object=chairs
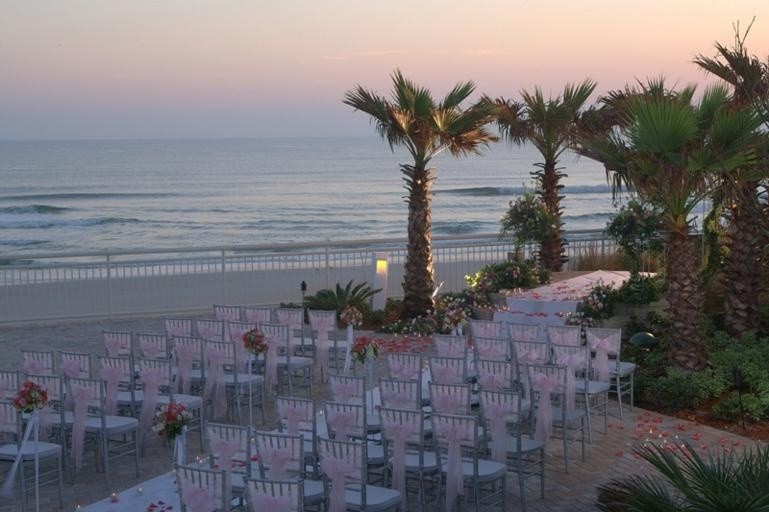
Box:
[355,318,643,512]
[0,299,354,511]
